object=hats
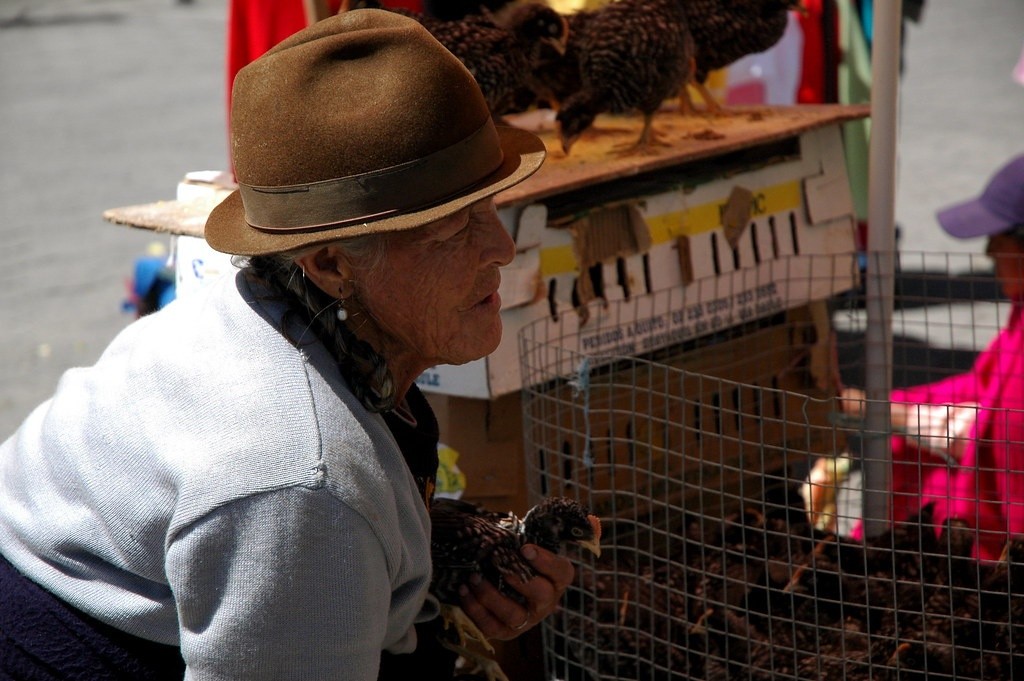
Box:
[937,155,1024,239]
[203,8,547,257]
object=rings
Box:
[509,612,529,630]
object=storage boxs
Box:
[425,300,854,527]
[171,123,866,399]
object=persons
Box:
[0,8,573,681]
[843,153,1024,567]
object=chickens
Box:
[339,1,1024,681]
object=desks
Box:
[96,99,870,243]
